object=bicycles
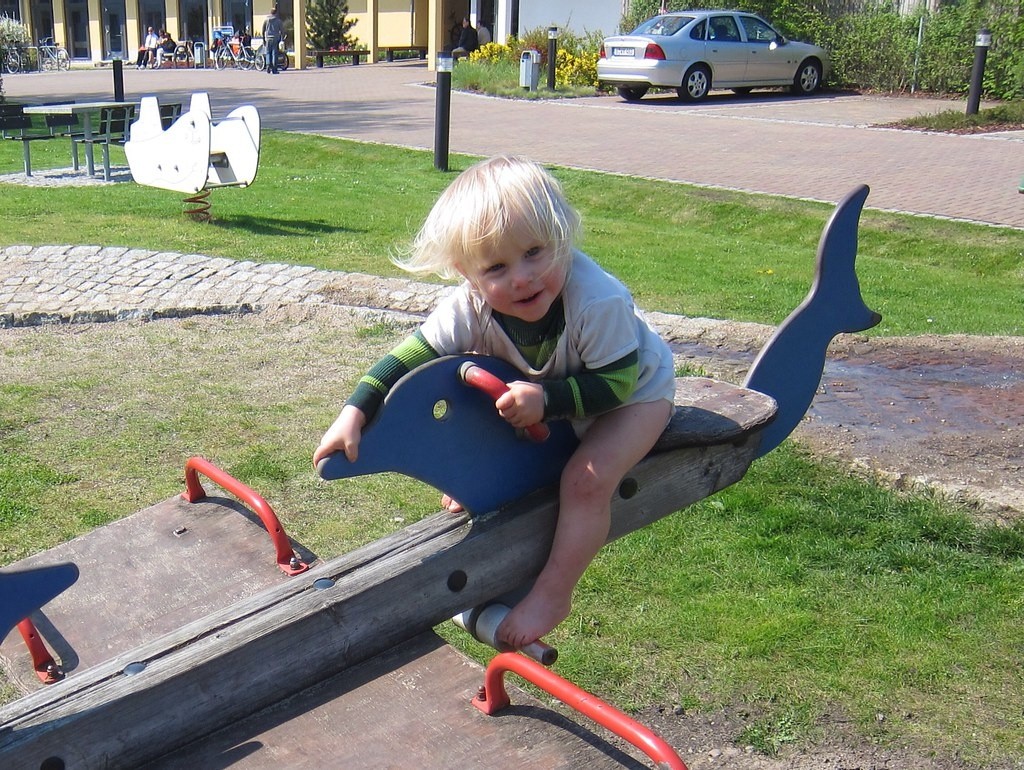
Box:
[0,32,32,75]
[38,35,72,72]
[214,32,256,71]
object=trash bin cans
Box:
[518,50,542,93]
[193,41,207,69]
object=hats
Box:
[162,33,170,38]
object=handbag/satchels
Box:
[279,41,285,53]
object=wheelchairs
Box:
[254,32,289,71]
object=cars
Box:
[596,10,830,105]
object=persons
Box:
[261,8,286,74]
[451,17,478,59]
[476,20,491,52]
[135,27,171,70]
[314,157,677,646]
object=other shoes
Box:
[267,66,270,73]
[272,70,279,74]
[140,65,145,70]
[135,65,139,69]
